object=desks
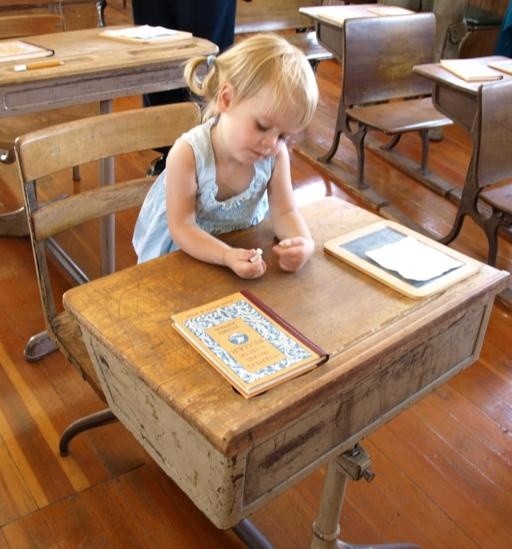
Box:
[298,4,415,61]
[375,55,510,244]
[0,24,219,284]
[62,198,510,548]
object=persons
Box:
[130,29,324,281]
[129,1,253,177]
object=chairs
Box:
[458,15,511,58]
[440,83,511,267]
[234,0,338,62]
[0,0,80,238]
[316,15,456,210]
[15,103,202,457]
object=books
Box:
[319,8,378,25]
[170,287,331,401]
[0,39,55,63]
[439,57,504,82]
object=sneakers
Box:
[145,155,165,175]
